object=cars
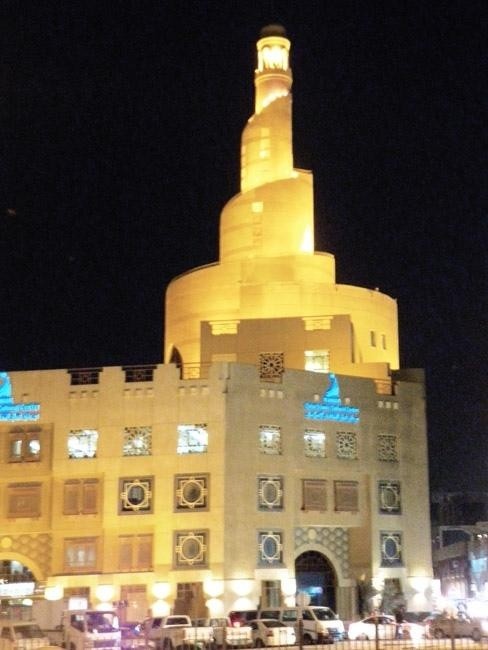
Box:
[243,618,296,648]
[117,625,156,649]
[346,612,487,644]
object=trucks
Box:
[190,615,254,650]
[134,614,216,650]
[29,592,124,649]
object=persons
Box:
[395,607,405,639]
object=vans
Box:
[0,617,49,650]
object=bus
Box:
[227,602,346,646]
